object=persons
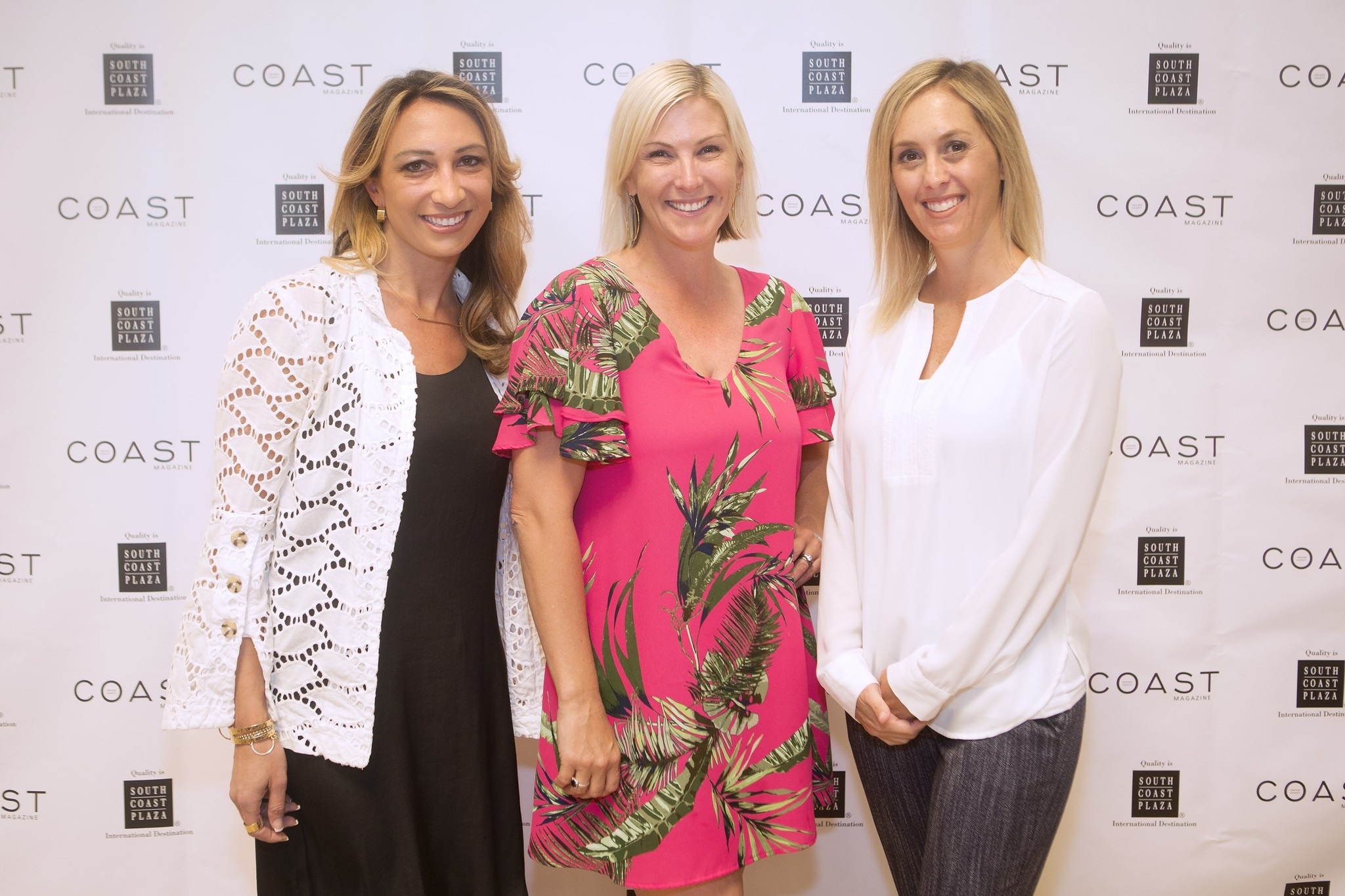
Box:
[814,60,1122,896]
[492,61,835,896]
[161,71,546,896]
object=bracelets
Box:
[219,718,283,755]
[813,532,822,544]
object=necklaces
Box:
[380,274,462,327]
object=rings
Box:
[571,777,590,788]
[244,819,261,833]
[798,554,814,568]
